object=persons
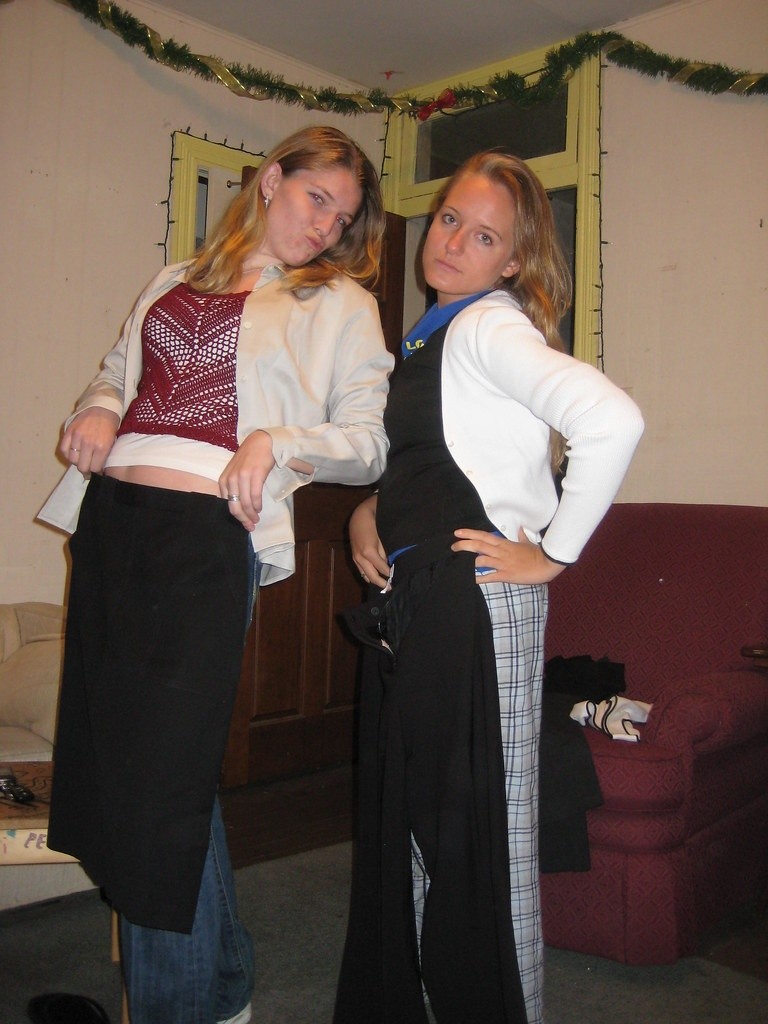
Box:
[36,122,397,1024]
[332,146,644,1024]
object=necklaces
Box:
[242,265,266,274]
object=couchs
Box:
[539,502,768,970]
[0,601,66,761]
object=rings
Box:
[227,494,239,502]
[361,574,366,578]
[70,447,80,451]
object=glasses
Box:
[0,773,37,805]
